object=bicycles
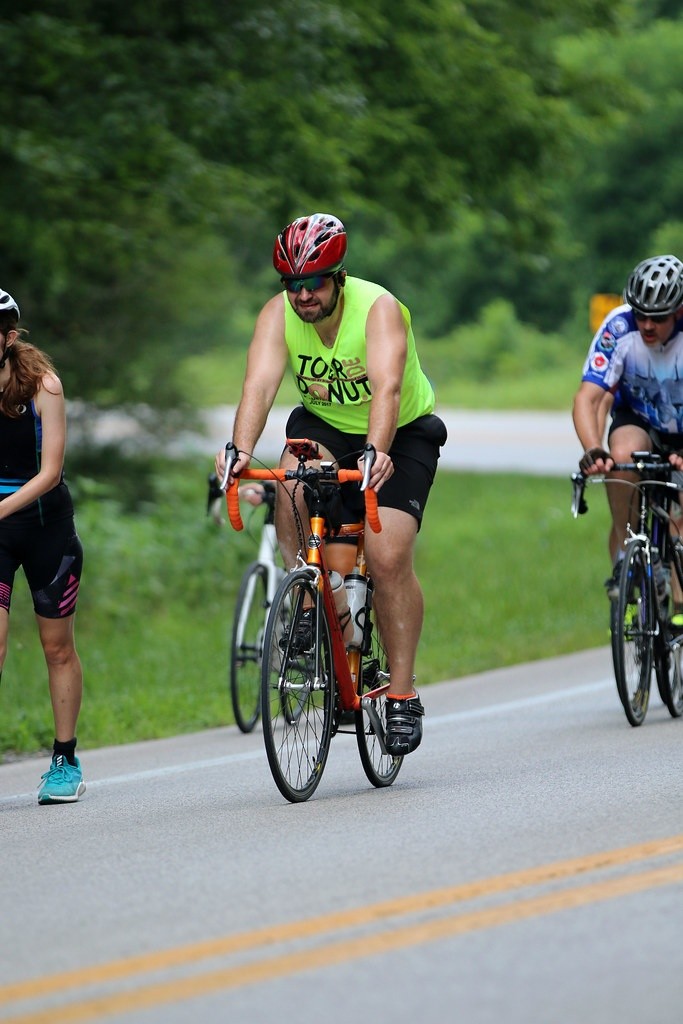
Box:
[203,470,320,737]
[566,450,683,727]
[218,437,406,805]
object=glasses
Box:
[280,273,333,292]
[635,312,673,322]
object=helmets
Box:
[626,255,683,316]
[0,289,20,325]
[274,213,347,278]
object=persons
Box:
[572,255,683,630]
[240,476,325,653]
[0,286,86,805]
[215,213,448,755]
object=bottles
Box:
[651,552,666,596]
[324,572,355,642]
[341,573,368,646]
[661,562,671,594]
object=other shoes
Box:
[606,561,633,598]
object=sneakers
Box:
[385,689,425,755]
[37,756,87,802]
[279,610,316,650]
